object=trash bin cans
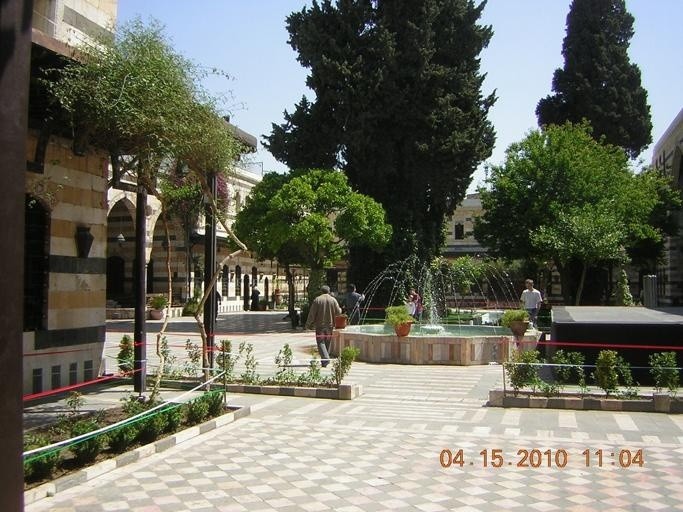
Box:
[268,302,275,310]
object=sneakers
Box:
[321,361,330,367]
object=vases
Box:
[336,313,348,329]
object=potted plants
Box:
[257,299,266,310]
[499,309,531,336]
[385,305,415,337]
[150,296,167,319]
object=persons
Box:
[520,279,543,327]
[347,283,365,325]
[306,285,342,367]
[402,288,424,321]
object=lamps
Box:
[75,225,94,259]
